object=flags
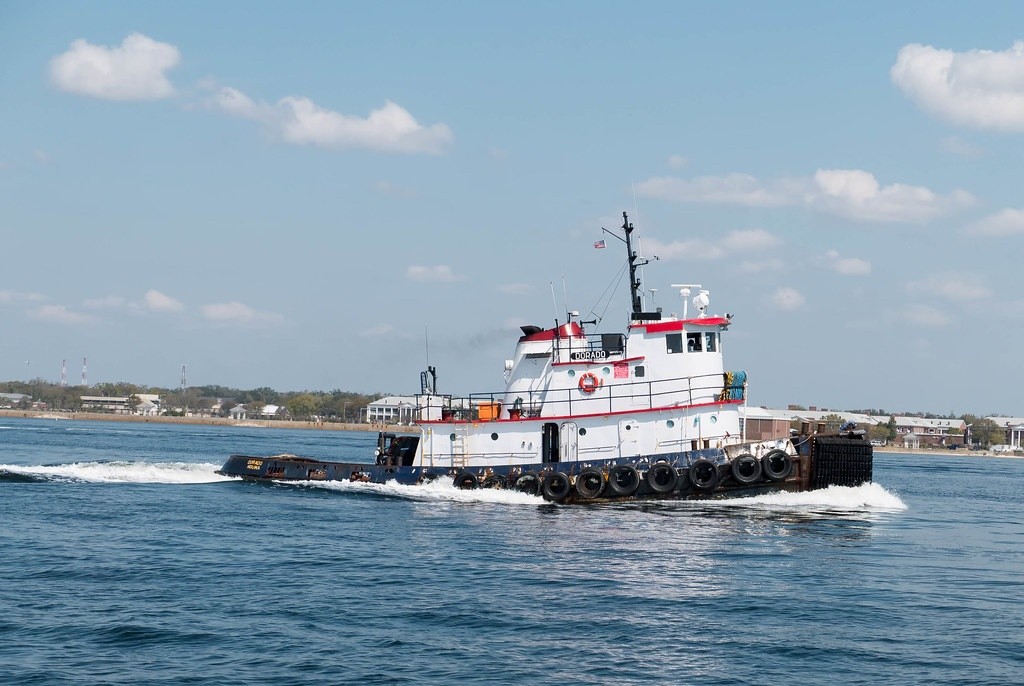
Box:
[592,240,606,249]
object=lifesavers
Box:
[578,372,599,393]
[451,471,571,501]
[688,458,720,491]
[575,464,640,500]
[646,462,679,495]
[730,448,793,485]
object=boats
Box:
[212,210,874,510]
